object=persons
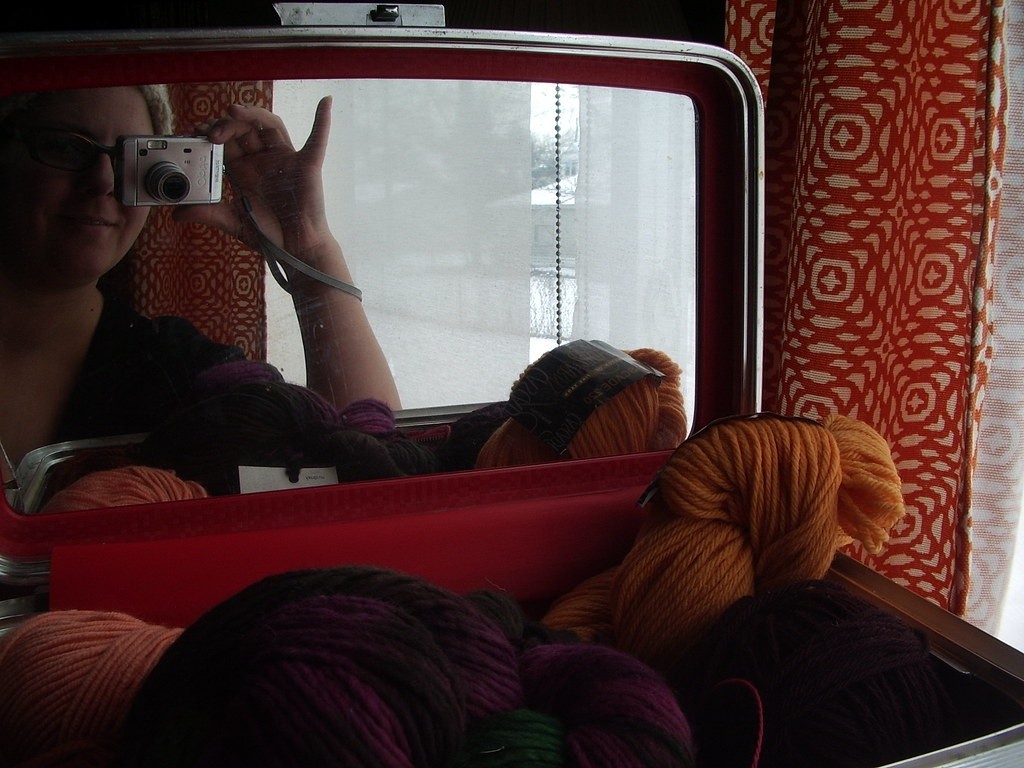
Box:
[1,83,401,465]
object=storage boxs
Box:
[0,2,1024,768]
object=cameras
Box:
[116,134,225,206]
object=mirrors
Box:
[2,78,696,515]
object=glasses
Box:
[9,123,117,173]
